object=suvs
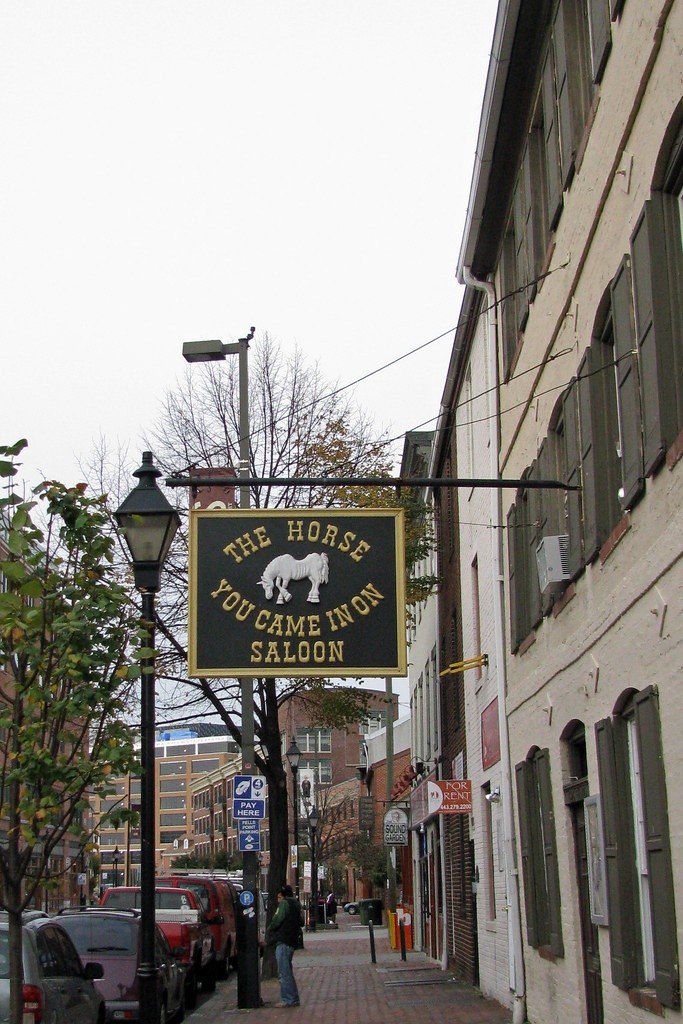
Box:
[51,906,186,1024]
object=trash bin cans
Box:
[387,910,413,949]
[357,899,383,925]
[308,898,327,924]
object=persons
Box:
[266,885,304,1007]
[324,890,338,924]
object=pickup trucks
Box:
[87,887,225,1011]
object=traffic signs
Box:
[238,819,261,852]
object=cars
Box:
[0,910,110,1024]
[344,901,360,915]
[211,878,241,973]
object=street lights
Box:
[183,326,258,889]
[112,846,121,886]
[284,734,304,903]
[109,450,182,1024]
[308,805,320,933]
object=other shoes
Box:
[274,1000,300,1008]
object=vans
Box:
[154,876,244,981]
